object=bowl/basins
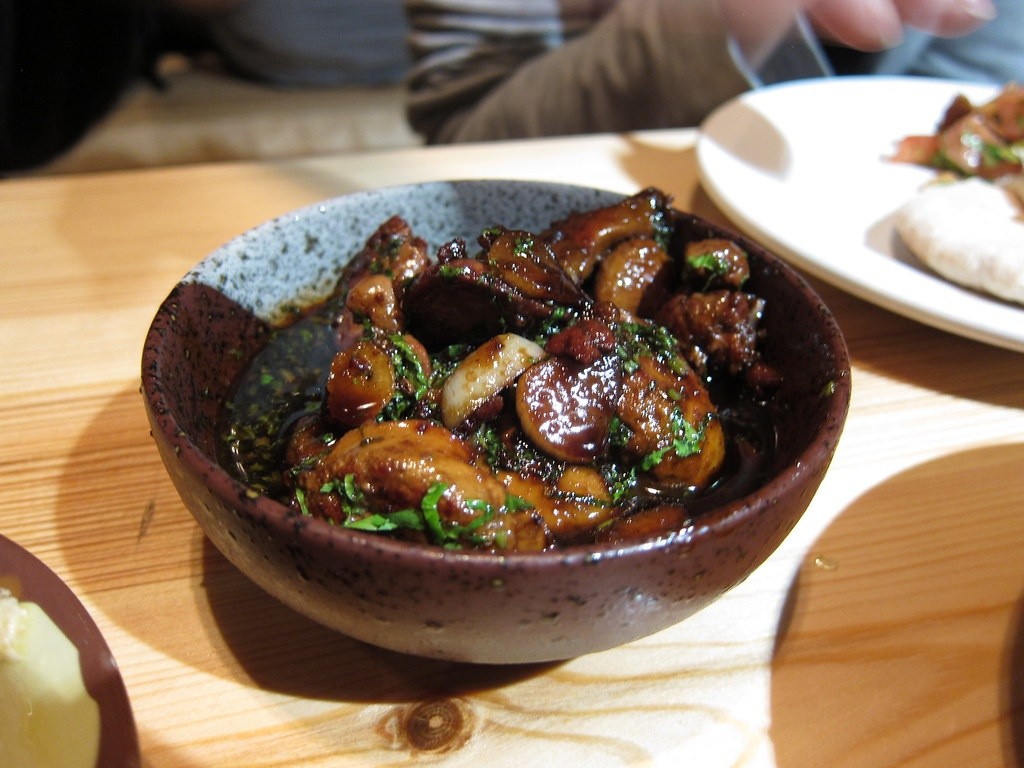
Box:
[140,180,852,666]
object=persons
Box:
[403,0,1024,147]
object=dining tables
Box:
[0,123,1024,768]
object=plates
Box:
[0,534,140,768]
[700,73,1024,354]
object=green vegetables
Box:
[299,318,710,552]
